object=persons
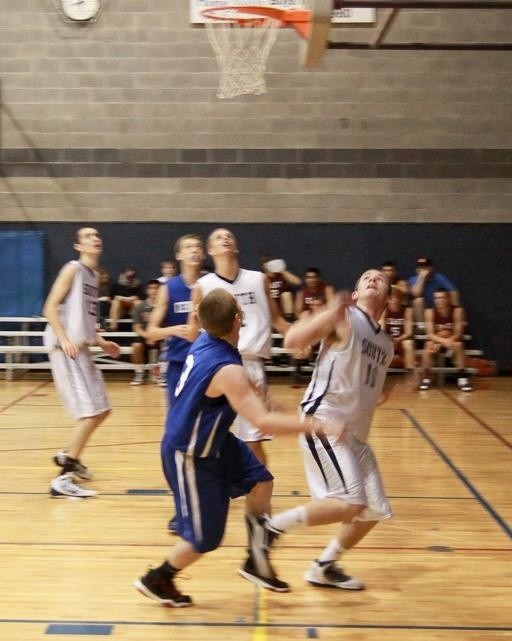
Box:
[131,280,162,385]
[263,255,304,324]
[290,297,326,388]
[106,267,138,332]
[418,288,472,391]
[246,270,392,589]
[156,260,177,386]
[291,269,337,319]
[146,234,211,531]
[94,267,112,333]
[135,290,353,606]
[185,228,290,468]
[42,226,122,500]
[378,288,418,387]
[382,262,410,305]
[407,258,461,335]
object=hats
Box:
[410,256,430,267]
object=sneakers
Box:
[167,516,181,534]
[53,452,92,480]
[237,555,290,592]
[157,374,169,386]
[304,559,364,590]
[130,374,145,386]
[134,569,192,607]
[247,514,275,556]
[458,378,473,391]
[419,376,433,390]
[50,477,98,500]
